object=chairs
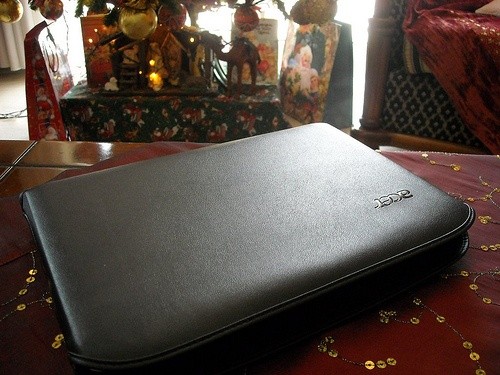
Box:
[347,0,499,155]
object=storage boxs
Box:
[79,13,121,87]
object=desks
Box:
[60,80,283,149]
[0,137,500,375]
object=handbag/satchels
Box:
[278,17,353,129]
[22,20,72,141]
[19,121,474,375]
[229,16,279,85]
